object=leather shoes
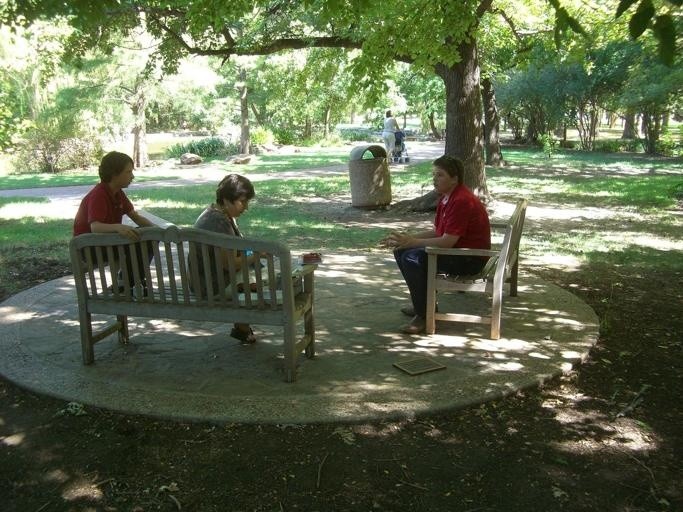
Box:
[399,301,439,333]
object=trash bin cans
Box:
[350,145,392,208]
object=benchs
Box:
[63,226,318,385]
[424,199,528,344]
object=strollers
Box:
[390,131,410,163]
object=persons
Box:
[74,152,160,296]
[382,111,400,163]
[187,174,266,344]
[380,155,491,334]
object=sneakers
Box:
[230,328,256,343]
